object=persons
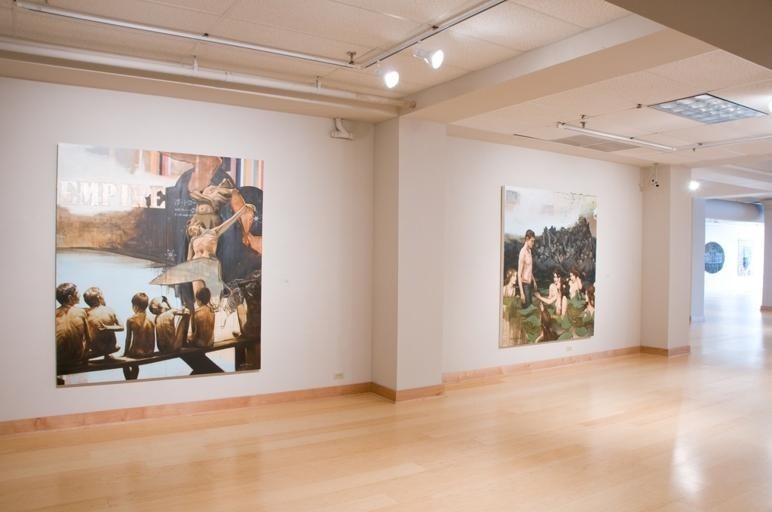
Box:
[81,287,125,363]
[121,292,157,380]
[149,294,191,354]
[503,267,519,347]
[186,203,257,310]
[170,156,255,310]
[517,228,539,307]
[534,267,594,343]
[187,287,216,348]
[56,282,92,385]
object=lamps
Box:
[376,60,399,88]
[413,40,444,69]
